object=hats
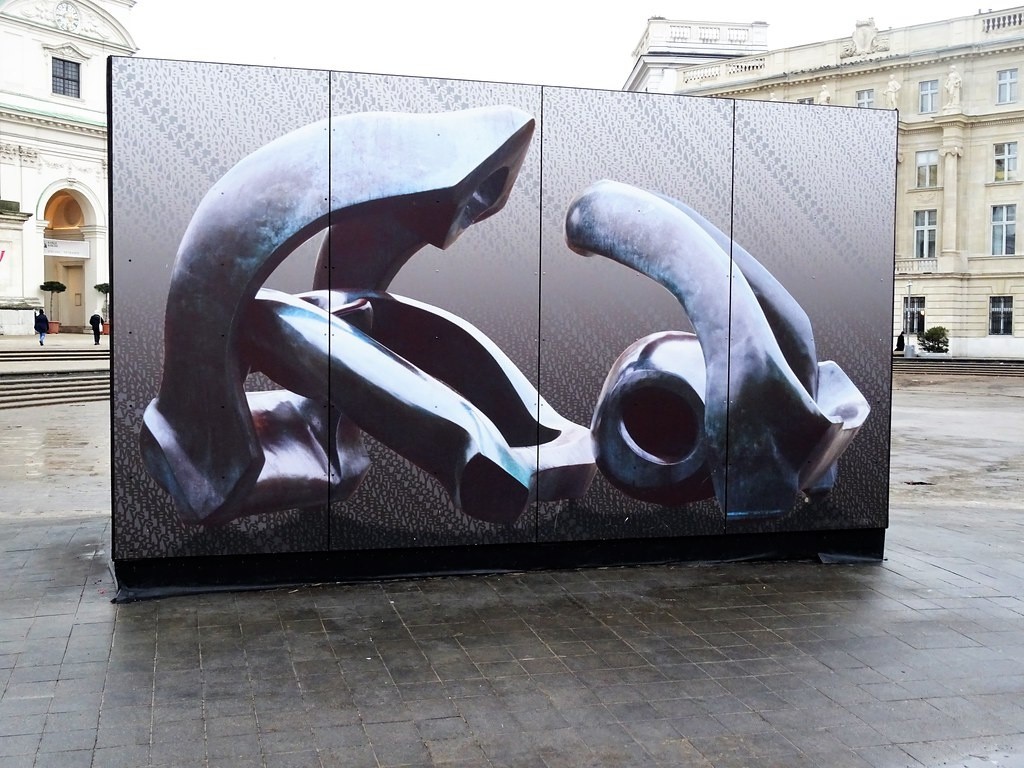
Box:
[39,309,44,313]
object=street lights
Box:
[906,280,913,345]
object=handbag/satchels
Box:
[99,317,103,332]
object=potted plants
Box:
[40,281,67,334]
[94,283,109,335]
[916,326,952,358]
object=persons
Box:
[944,65,962,109]
[90,311,104,345]
[818,85,830,104]
[897,331,904,357]
[769,93,777,101]
[34,309,49,345]
[883,74,901,109]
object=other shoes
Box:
[40,341,43,346]
[95,342,99,345]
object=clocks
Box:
[54,0,80,32]
